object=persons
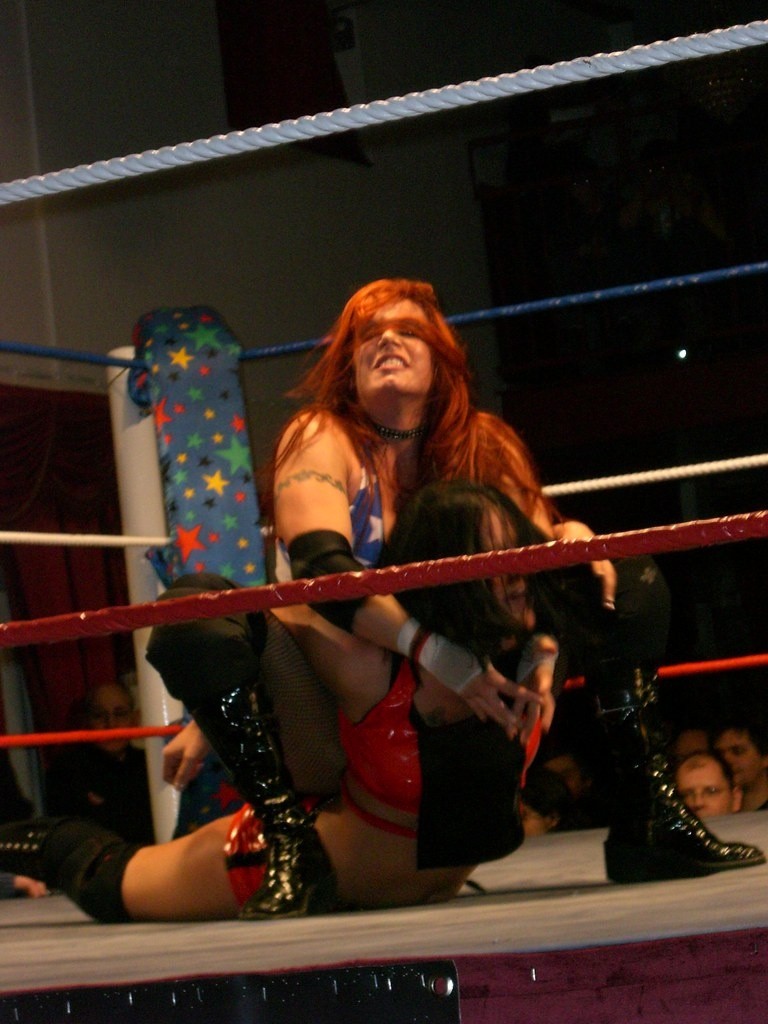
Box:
[0,479,768,923]
[143,276,768,916]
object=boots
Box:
[173,677,338,923]
[577,658,765,886]
[0,814,74,881]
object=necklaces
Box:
[364,419,429,443]
